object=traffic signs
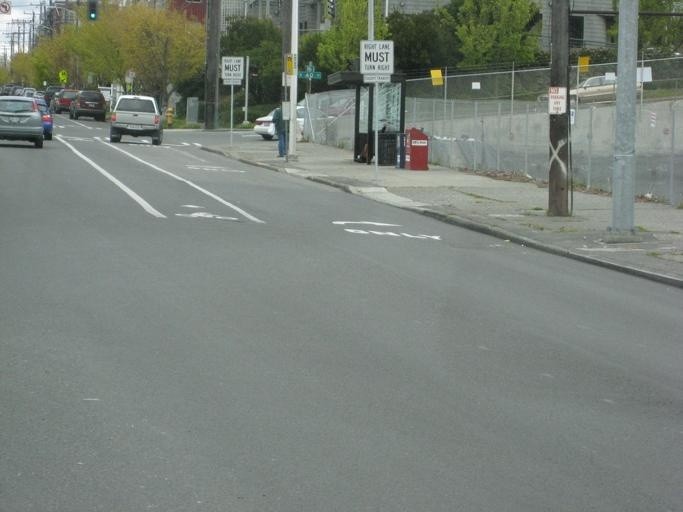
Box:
[298,61,321,80]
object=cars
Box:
[254,105,338,140]
[536,75,643,105]
[109,94,163,145]
[0,83,106,148]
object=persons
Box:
[272,100,290,158]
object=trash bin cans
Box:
[377,130,397,167]
[357,133,375,162]
[403,128,428,170]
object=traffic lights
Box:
[88,2,97,19]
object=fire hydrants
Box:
[165,106,174,129]
